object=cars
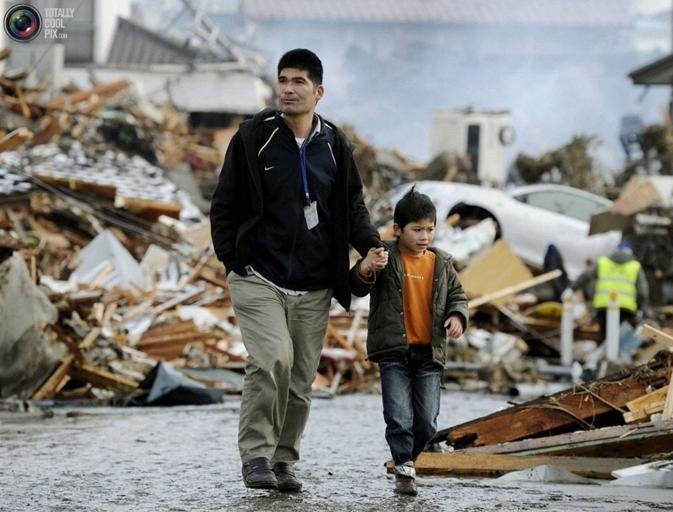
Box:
[375,174,668,304]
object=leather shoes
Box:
[242,457,279,489]
[274,461,302,492]
[392,461,417,495]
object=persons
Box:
[349,183,469,497]
[591,240,651,342]
[651,264,672,329]
[209,45,391,496]
[569,255,598,316]
